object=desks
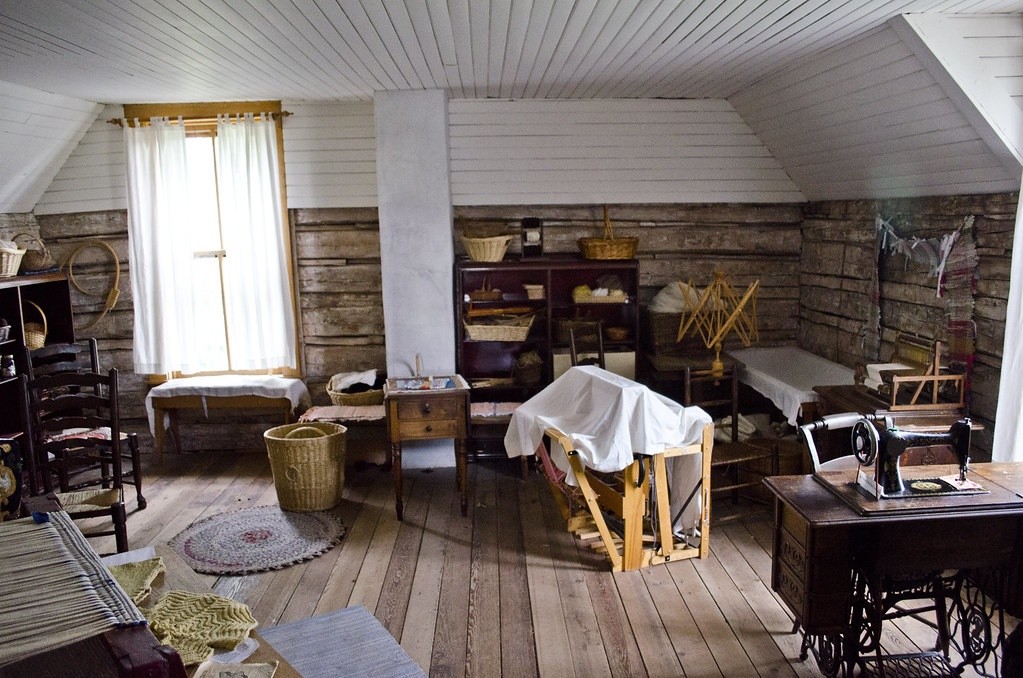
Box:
[384,371,472,523]
[722,344,856,473]
[757,457,1023,678]
[143,373,308,463]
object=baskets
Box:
[553,317,604,343]
[577,203,639,261]
[11,232,50,271]
[458,234,514,263]
[0,243,26,277]
[463,315,535,342]
[21,299,49,351]
[515,360,543,386]
[326,377,385,406]
[571,292,629,303]
[606,326,633,340]
[469,272,502,301]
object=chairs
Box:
[25,335,148,512]
[684,360,780,532]
[14,366,129,554]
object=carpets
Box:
[165,503,348,578]
[256,603,429,678]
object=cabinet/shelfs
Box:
[0,271,81,478]
[454,252,640,468]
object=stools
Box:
[796,407,956,664]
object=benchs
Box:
[100,541,303,678]
[297,401,531,478]
